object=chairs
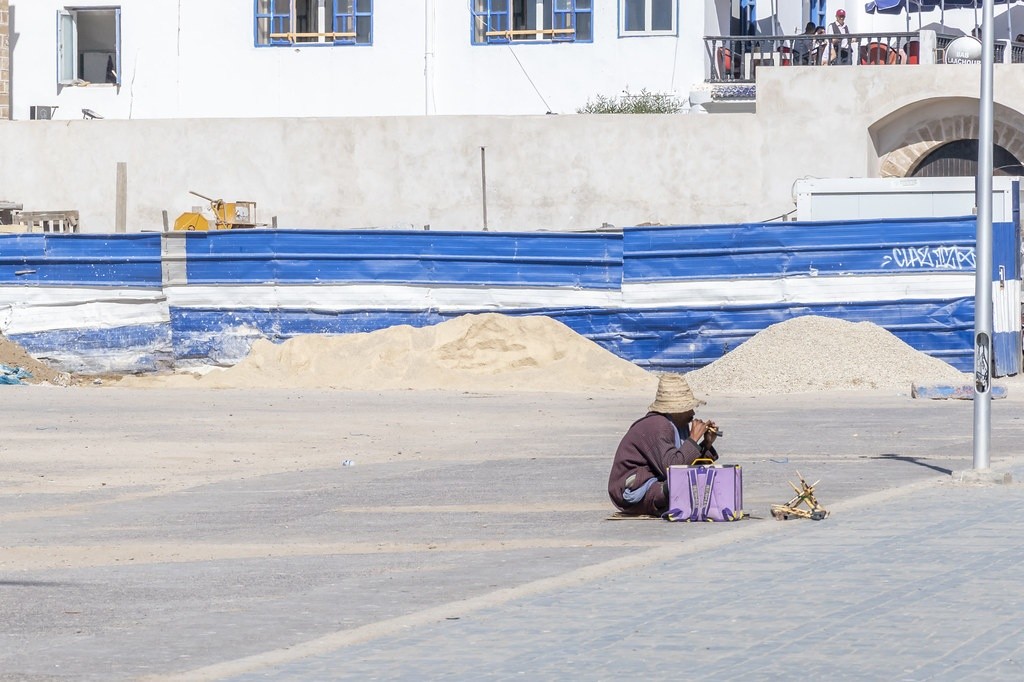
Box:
[860,41,919,64]
[777,46,800,65]
[717,47,742,78]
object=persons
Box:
[792,22,816,66]
[827,9,857,65]
[1016,34,1024,43]
[971,28,982,40]
[608,373,719,517]
[809,26,837,65]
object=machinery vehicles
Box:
[190,190,257,230]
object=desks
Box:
[744,52,794,80]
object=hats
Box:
[650,373,706,413]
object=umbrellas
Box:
[865,0,1024,42]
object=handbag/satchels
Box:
[662,458,744,521]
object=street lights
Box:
[937,34,994,472]
